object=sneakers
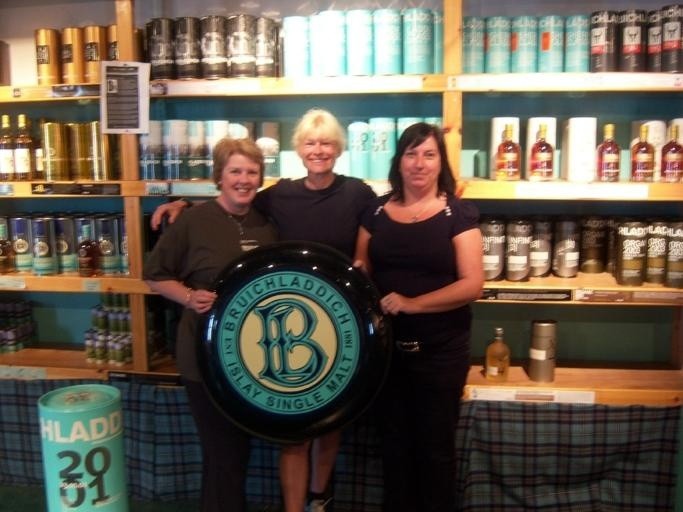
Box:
[306,490,331,512]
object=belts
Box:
[395,321,470,353]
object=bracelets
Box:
[180,198,194,207]
[183,288,192,305]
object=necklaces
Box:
[403,198,436,223]
[227,210,250,236]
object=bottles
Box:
[529,125,555,183]
[596,123,621,181]
[1,114,49,179]
[0,239,15,275]
[630,122,656,185]
[76,225,102,276]
[483,326,512,384]
[660,124,683,183]
[495,121,522,181]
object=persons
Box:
[354,123,484,512]
[152,110,383,512]
[142,138,278,512]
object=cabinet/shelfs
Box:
[121,1,449,402]
[453,2,680,410]
[0,0,139,378]
